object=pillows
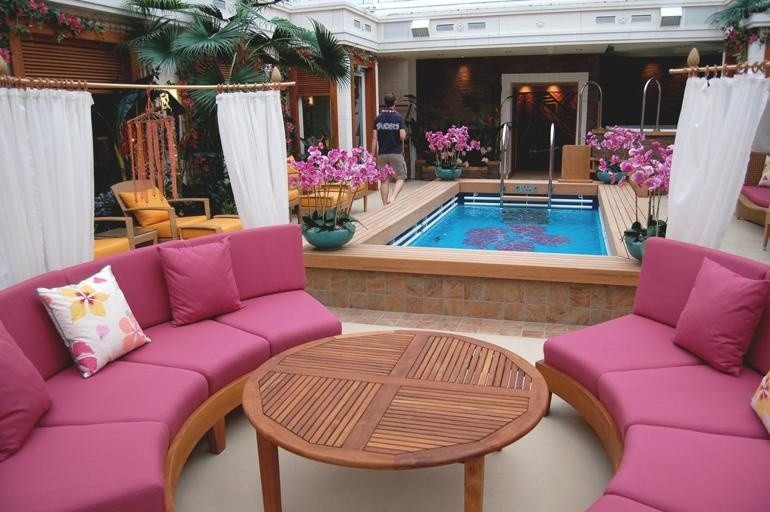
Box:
[671,257,770,378]
[750,374,769,436]
[759,155,770,186]
[35,265,151,379]
[156,235,246,326]
[1,322,54,461]
[118,187,174,227]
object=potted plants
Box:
[484,82,519,179]
[705,0,770,29]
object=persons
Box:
[371,95,408,207]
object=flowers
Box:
[287,141,395,230]
[617,141,674,243]
[582,124,646,184]
[425,124,481,171]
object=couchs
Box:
[0,223,343,512]
[737,186,770,247]
[534,237,769,512]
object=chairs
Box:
[110,178,241,249]
[94,217,135,259]
[288,154,369,224]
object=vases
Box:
[624,238,643,262]
[301,223,356,250]
[435,167,463,181]
[597,170,628,184]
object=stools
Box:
[96,226,159,246]
